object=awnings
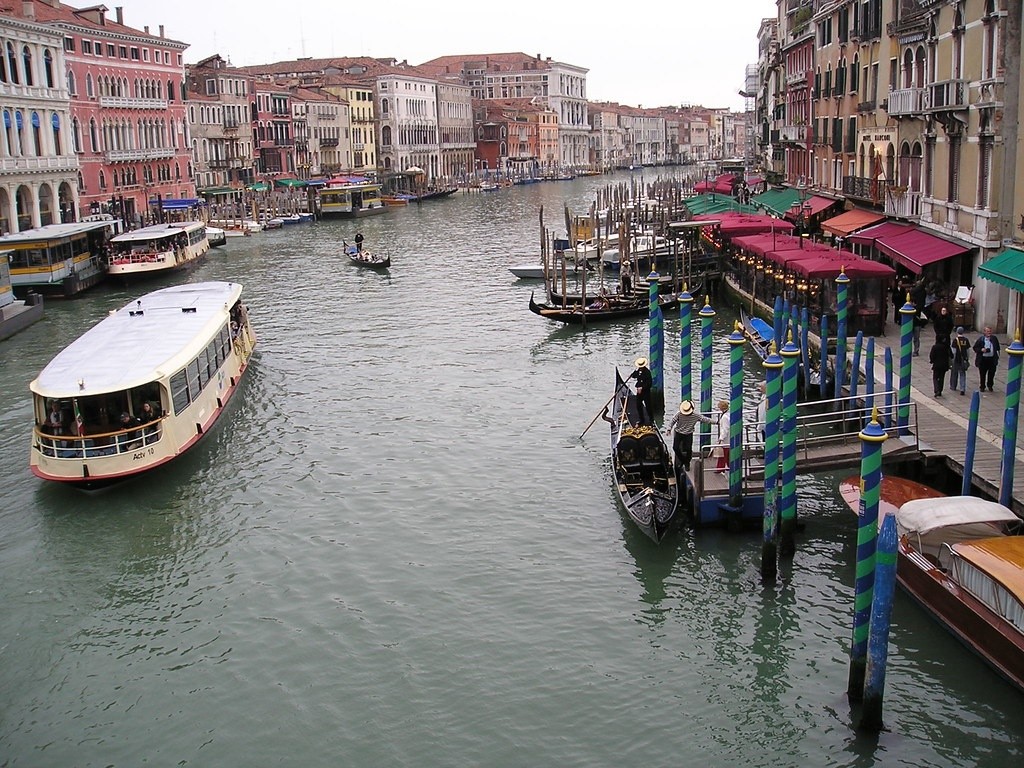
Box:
[746,178,764,185]
[977,248,1024,294]
[750,188,981,275]
[200,179,326,195]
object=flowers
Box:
[889,186,906,198]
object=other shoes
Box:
[677,457,685,468]
[933,391,941,397]
[685,463,690,471]
[960,389,964,395]
[714,469,725,473]
[980,386,985,392]
[950,387,956,390]
[988,385,993,392]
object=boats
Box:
[28,278,259,491]
[206,205,321,236]
[281,187,321,211]
[507,186,704,323]
[608,365,679,547]
[838,472,1024,696]
[429,164,602,192]
[0,218,123,300]
[105,221,210,278]
[205,224,226,248]
[319,184,389,218]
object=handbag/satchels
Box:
[961,358,969,371]
[712,441,725,458]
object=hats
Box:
[635,358,648,368]
[680,400,694,415]
[957,327,964,334]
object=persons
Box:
[666,400,718,471]
[713,401,730,473]
[45,401,67,449]
[355,231,364,251]
[92,234,188,262]
[631,357,654,426]
[120,399,161,451]
[231,300,241,340]
[757,380,766,460]
[891,277,1001,397]
[620,261,632,298]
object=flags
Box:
[869,154,883,206]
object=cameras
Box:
[985,348,991,353]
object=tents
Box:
[682,170,795,249]
[406,166,422,171]
[327,176,370,185]
[729,236,896,336]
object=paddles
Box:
[579,369,636,439]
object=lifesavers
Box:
[138,256,150,263]
